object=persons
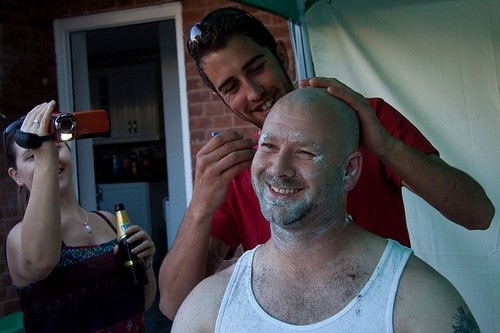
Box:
[158,6,496,321]
[171,86,482,333]
[3,100,156,333]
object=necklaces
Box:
[60,207,93,233]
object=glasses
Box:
[187,7,259,61]
[3,115,26,168]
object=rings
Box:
[32,120,40,125]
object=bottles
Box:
[113,204,150,295]
[111,147,118,169]
[131,152,138,174]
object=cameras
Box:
[47,109,110,145]
